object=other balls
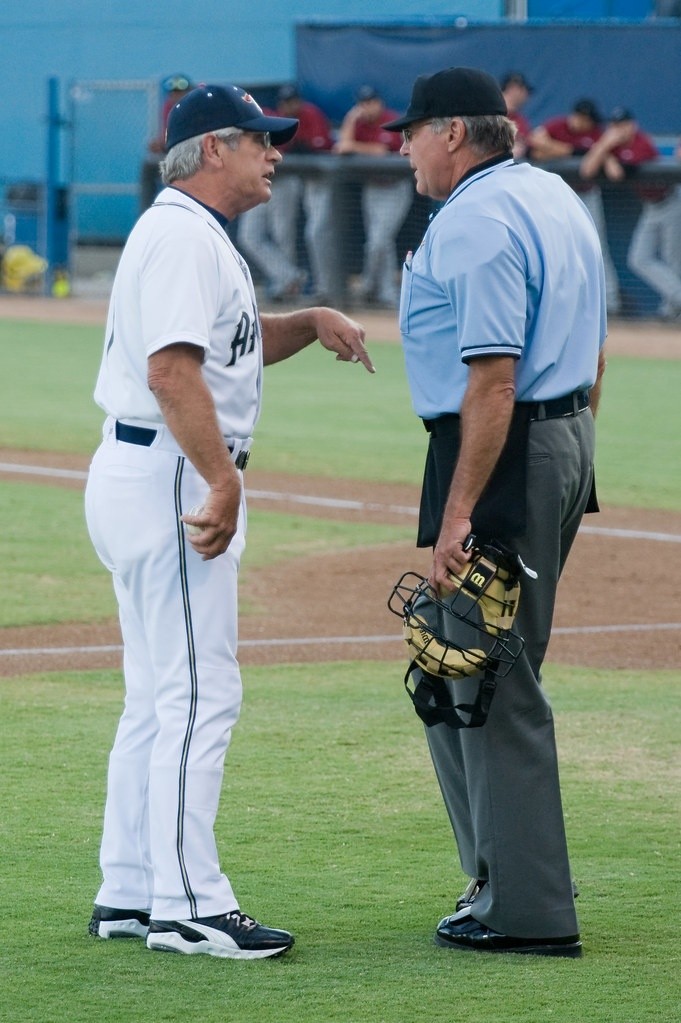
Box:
[53,280,68,297]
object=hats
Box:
[163,83,300,151]
[268,84,297,100]
[500,70,534,93]
[608,104,636,122]
[379,67,508,131]
[356,84,377,102]
[574,99,604,122]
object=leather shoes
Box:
[455,878,488,912]
[436,907,583,957]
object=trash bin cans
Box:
[1,181,71,270]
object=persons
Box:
[86,84,374,960]
[501,71,681,320]
[148,73,415,306]
[382,69,609,957]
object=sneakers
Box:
[146,909,293,961]
[87,904,155,939]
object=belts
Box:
[422,391,591,431]
[116,420,250,469]
